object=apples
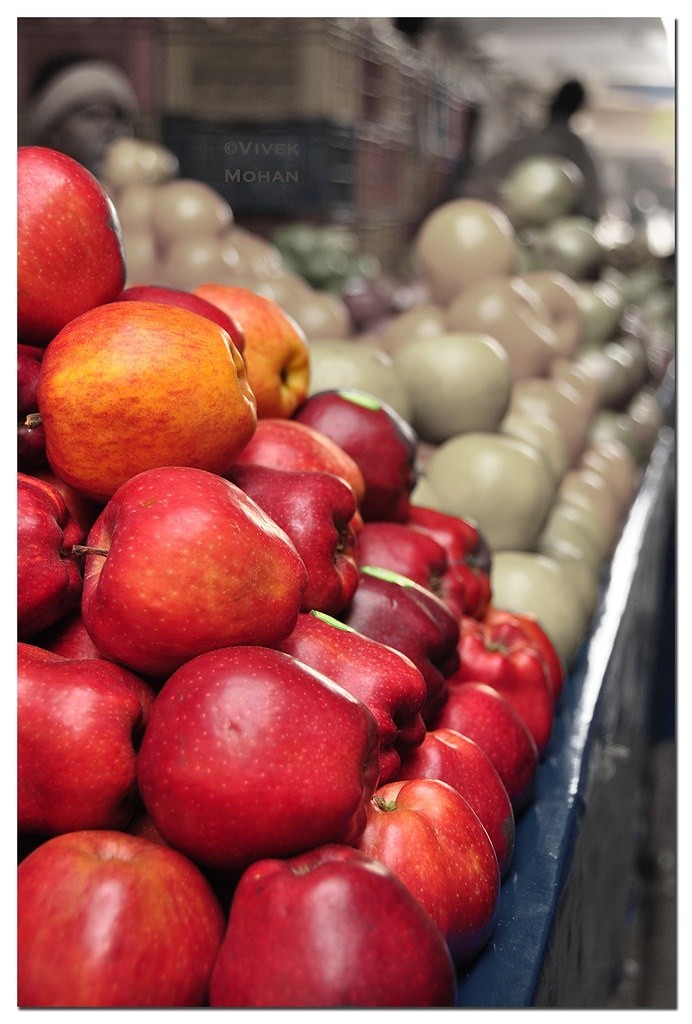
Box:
[83,138,675,671]
[17,147,561,1008]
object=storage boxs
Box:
[168,22,478,266]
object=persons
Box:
[462,77,597,221]
[22,48,139,177]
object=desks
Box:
[450,419,675,1011]
